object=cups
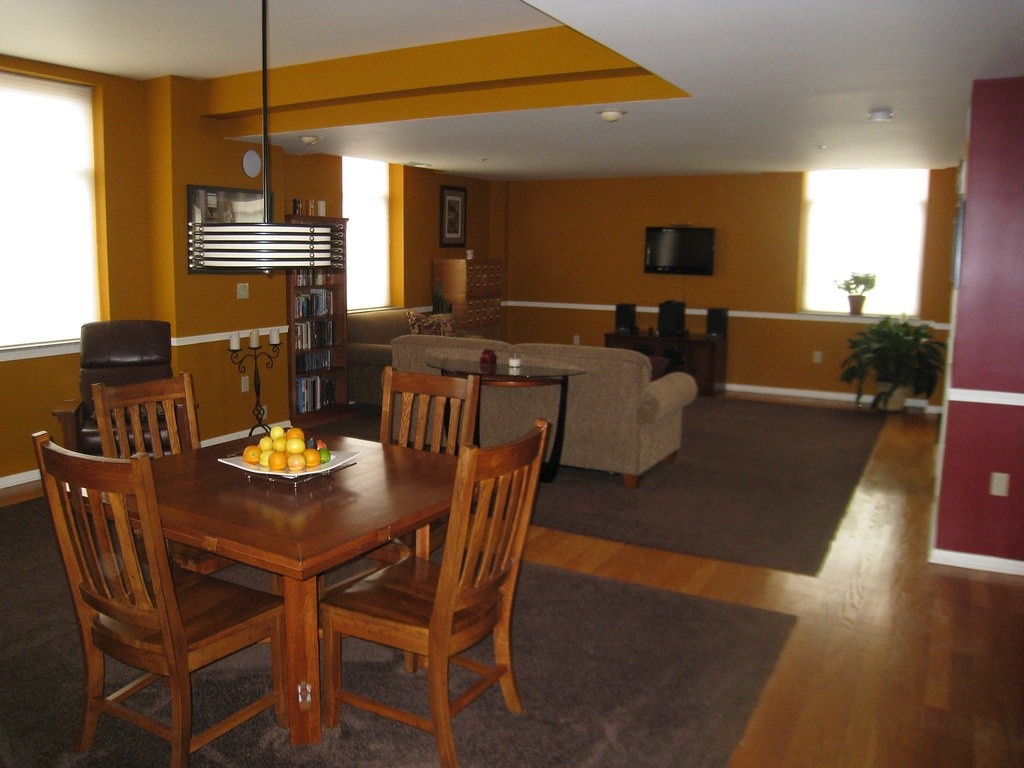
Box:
[466,250,474,259]
[317,201,325,216]
[306,200,316,216]
[509,352,521,368]
[293,198,302,215]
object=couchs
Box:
[384,331,703,489]
[347,306,492,410]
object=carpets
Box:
[0,491,801,768]
[483,395,892,576]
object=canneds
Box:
[317,200,326,216]
[293,198,303,215]
[306,199,315,216]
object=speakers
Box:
[707,308,728,337]
[659,301,685,336]
[615,304,636,332]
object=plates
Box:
[218,450,362,479]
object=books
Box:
[295,288,333,318]
[295,320,333,350]
[296,375,336,413]
[295,266,334,286]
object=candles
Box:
[228,331,241,349]
[247,329,261,347]
[269,327,280,344]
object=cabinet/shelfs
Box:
[287,214,350,418]
[429,257,504,328]
[599,329,729,398]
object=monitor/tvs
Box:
[644,227,716,275]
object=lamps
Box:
[594,108,628,124]
[298,135,320,146]
[865,111,896,123]
[187,0,334,276]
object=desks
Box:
[426,358,587,487]
[60,426,499,749]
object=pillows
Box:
[432,312,460,336]
[405,308,443,337]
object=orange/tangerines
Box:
[242,426,321,471]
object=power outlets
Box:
[989,472,1009,498]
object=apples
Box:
[317,440,330,462]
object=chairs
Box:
[320,416,551,768]
[48,317,186,461]
[91,369,203,460]
[380,367,482,557]
[30,429,289,768]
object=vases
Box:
[317,199,326,215]
[293,197,303,215]
[305,198,316,216]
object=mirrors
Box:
[188,184,278,275]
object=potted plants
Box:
[838,314,948,419]
[832,270,877,314]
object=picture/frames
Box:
[439,184,467,248]
